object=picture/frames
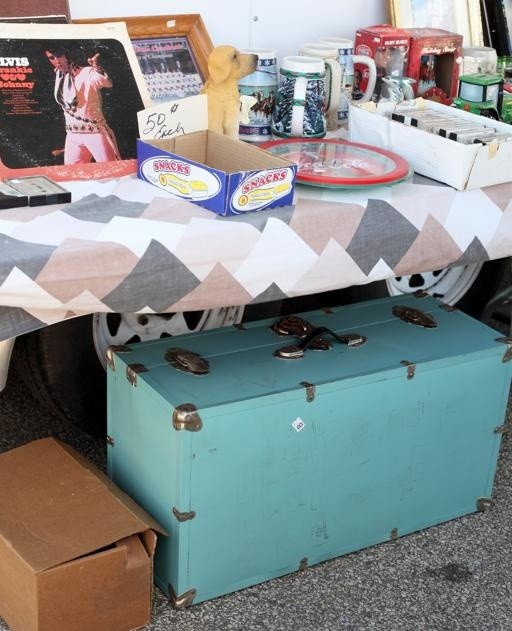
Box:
[70,13,215,106]
[388,0,485,48]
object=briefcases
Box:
[107,288,510,605]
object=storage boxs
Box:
[348,97,512,190]
[0,436,166,631]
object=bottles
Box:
[496,56,511,83]
[269,57,326,141]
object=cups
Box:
[320,35,377,128]
[238,48,277,142]
[461,47,497,76]
[298,44,342,116]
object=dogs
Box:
[197,45,259,140]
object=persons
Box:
[45,43,122,165]
